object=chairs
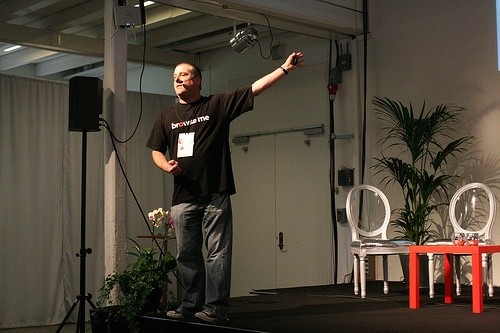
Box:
[429,182,493,301]
[346,184,415,299]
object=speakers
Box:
[68,76,104,132]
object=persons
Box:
[146,51,304,321]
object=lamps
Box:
[229,27,259,56]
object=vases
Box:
[119,282,165,307]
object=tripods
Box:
[55,132,98,333]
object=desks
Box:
[407,242,500,314]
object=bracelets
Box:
[279,65,288,75]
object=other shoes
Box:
[167,307,195,319]
[195,310,222,322]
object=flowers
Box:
[106,206,180,287]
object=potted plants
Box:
[362,95,466,296]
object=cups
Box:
[451,233,465,246]
[466,232,479,246]
